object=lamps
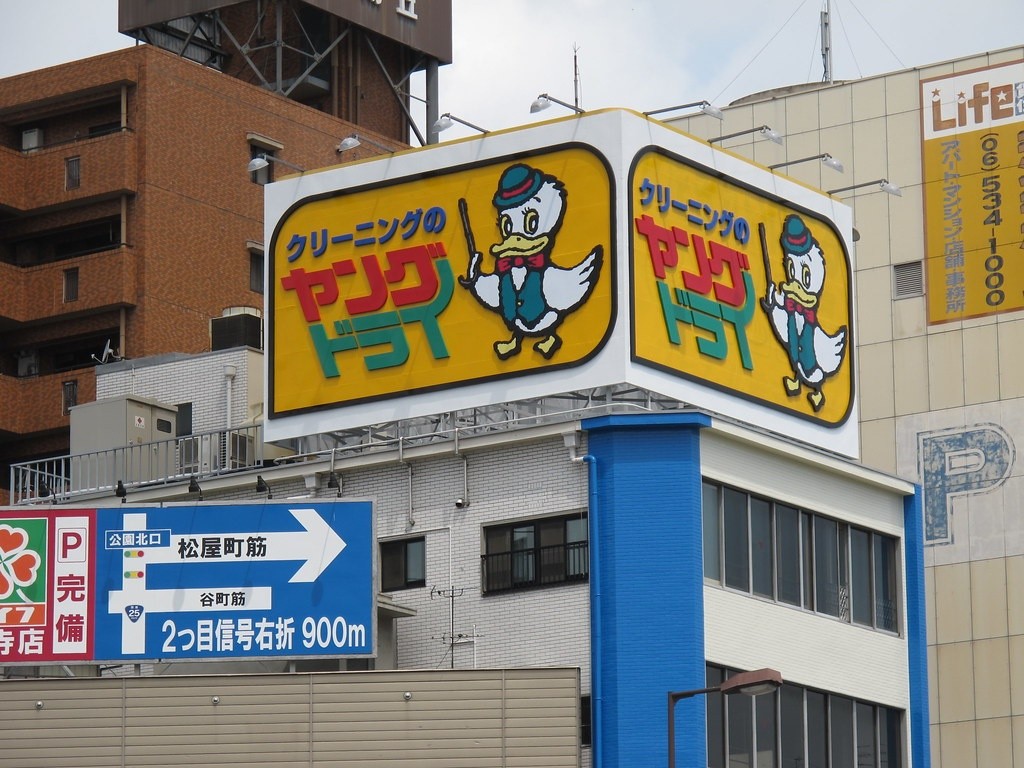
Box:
[339,132,395,154]
[530,93,585,114]
[327,471,346,498]
[247,153,305,173]
[116,480,127,503]
[39,480,57,505]
[256,476,273,500]
[826,179,902,197]
[707,124,783,146]
[642,99,724,120]
[189,476,203,501]
[431,113,490,134]
[768,152,844,174]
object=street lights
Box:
[669,667,783,768]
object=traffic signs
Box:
[94,500,381,661]
